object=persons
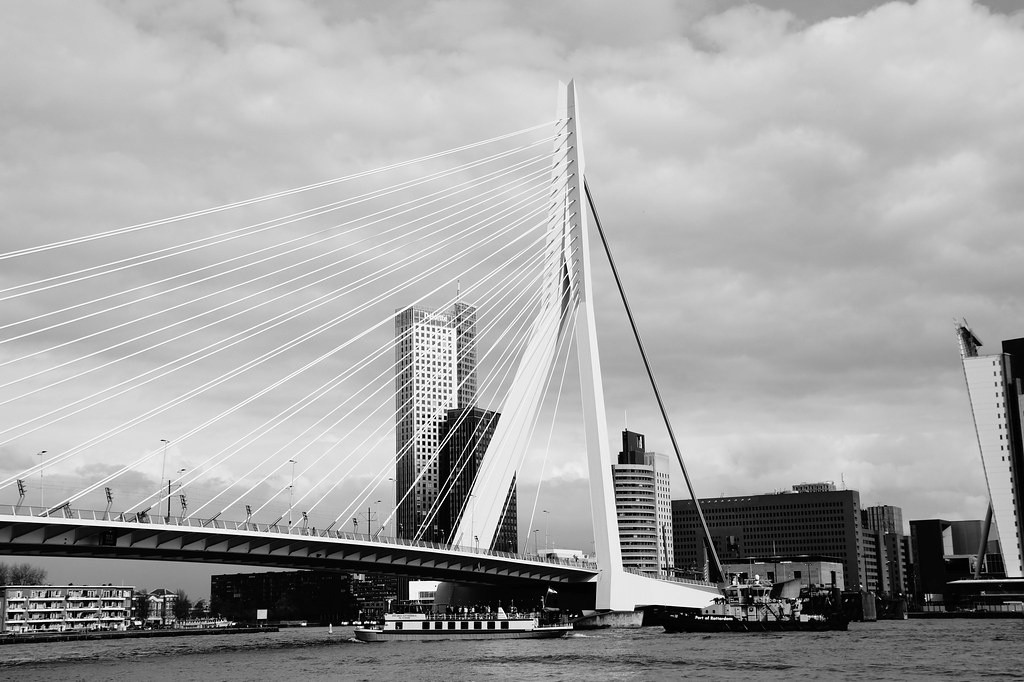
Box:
[445,604,491,620]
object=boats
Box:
[354,596,573,642]
[662,574,861,632]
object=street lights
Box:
[157,439,169,524]
[289,460,297,534]
[38,450,46,511]
[544,510,551,550]
[377,500,381,528]
[471,495,477,553]
[389,478,397,538]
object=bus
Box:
[944,579,1024,613]
